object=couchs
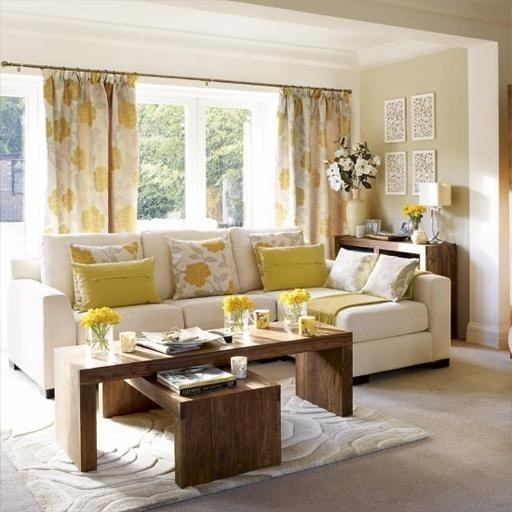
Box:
[1,229,452,399]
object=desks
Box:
[335,235,457,338]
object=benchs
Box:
[99,366,282,489]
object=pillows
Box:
[70,256,160,312]
[253,242,328,292]
[323,248,378,292]
[166,232,239,300]
[67,237,144,311]
[248,230,305,247]
[358,254,420,302]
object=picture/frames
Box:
[412,150,436,195]
[411,93,435,141]
[384,98,406,143]
[385,152,406,195]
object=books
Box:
[156,364,237,397]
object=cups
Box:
[249,309,271,329]
[299,315,316,337]
[231,355,250,378]
[119,330,137,353]
[355,223,366,239]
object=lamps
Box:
[419,183,451,244]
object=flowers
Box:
[81,307,120,350]
[323,137,380,192]
[401,204,427,230]
[223,296,255,329]
[280,288,311,321]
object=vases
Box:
[346,189,366,237]
[86,326,113,358]
[224,310,249,338]
[284,303,308,329]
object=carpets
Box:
[0,362,427,512]
